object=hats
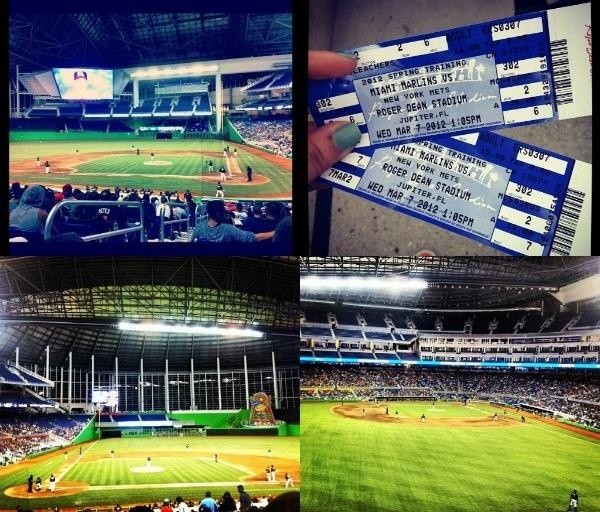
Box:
[74,71,87,80]
[62,184,73,191]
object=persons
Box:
[44,160,51,173]
[16,505,21,511]
[49,474,56,494]
[567,489,579,511]
[0,407,116,469]
[214,452,218,464]
[83,505,89,512]
[215,164,227,183]
[308,47,364,192]
[9,180,292,256]
[270,464,276,482]
[285,473,294,489]
[136,148,140,156]
[36,155,41,166]
[205,159,215,175]
[53,506,59,512]
[147,119,209,132]
[27,474,34,493]
[150,151,154,162]
[266,466,272,483]
[246,165,252,182]
[35,477,43,492]
[213,89,292,160]
[113,484,275,512]
[131,145,135,151]
[147,456,152,468]
